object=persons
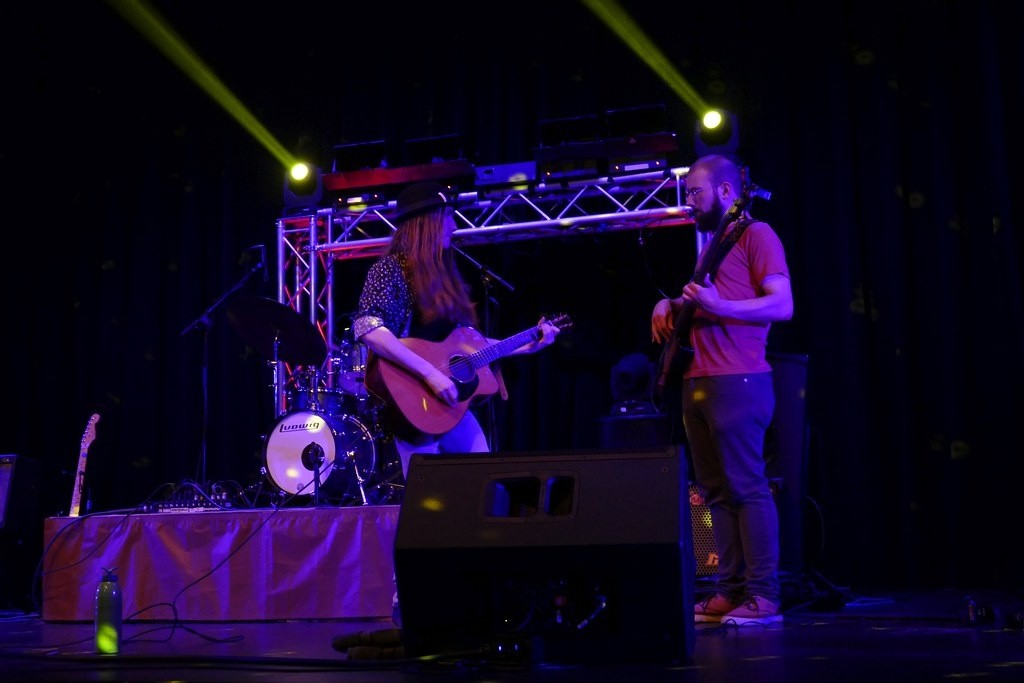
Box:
[354,181,560,480]
[652,155,794,625]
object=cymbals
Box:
[230,294,330,360]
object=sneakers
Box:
[694,595,738,622]
[722,595,782,625]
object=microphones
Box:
[309,444,320,465]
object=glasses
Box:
[686,182,724,200]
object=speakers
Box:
[393,443,697,668]
[0,454,43,540]
[758,350,809,599]
[684,479,720,578]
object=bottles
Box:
[94,565,123,654]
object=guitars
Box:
[67,413,101,516]
[367,312,574,436]
[652,185,760,411]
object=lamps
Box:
[288,165,328,204]
[699,108,732,146]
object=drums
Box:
[338,339,371,397]
[259,408,377,492]
[285,386,368,423]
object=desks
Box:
[42,502,396,621]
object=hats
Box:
[388,180,465,225]
